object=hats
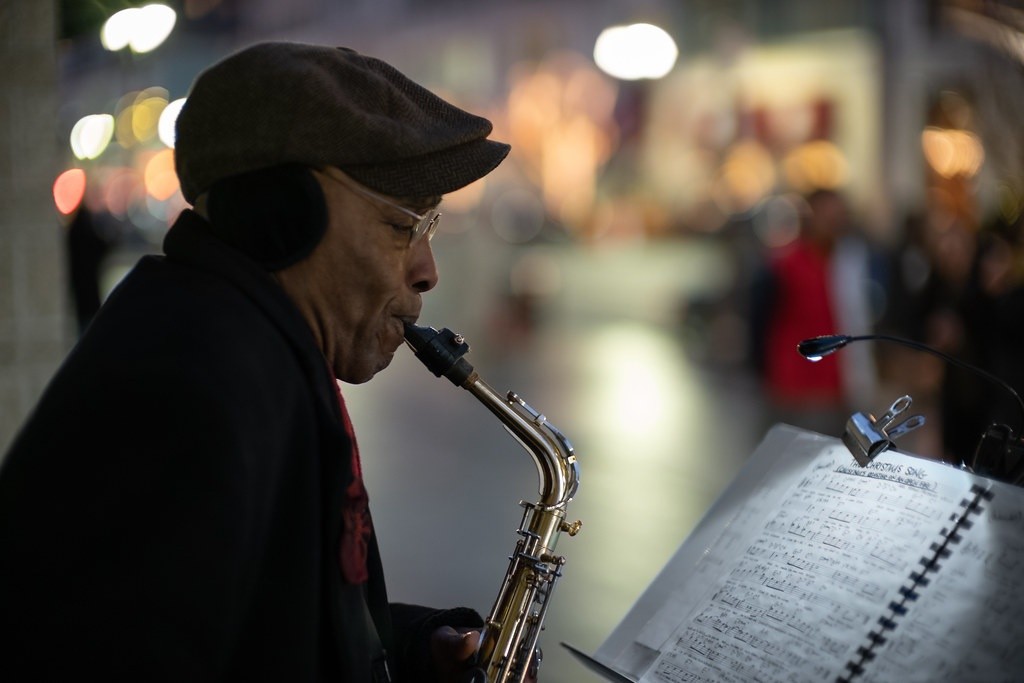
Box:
[174,40,511,200]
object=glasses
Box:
[305,163,442,250]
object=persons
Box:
[0,43,538,683]
[677,168,1024,487]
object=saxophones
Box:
[402,320,586,682]
